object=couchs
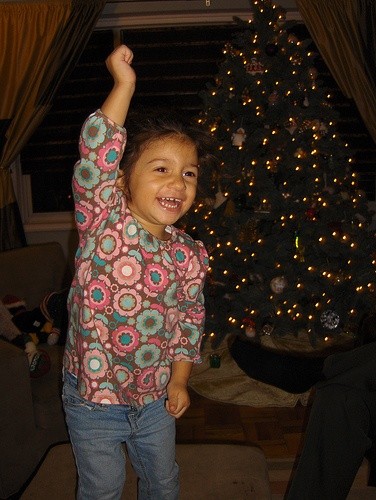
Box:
[0,242,68,500]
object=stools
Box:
[20,443,272,500]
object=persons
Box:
[61,44,211,499]
